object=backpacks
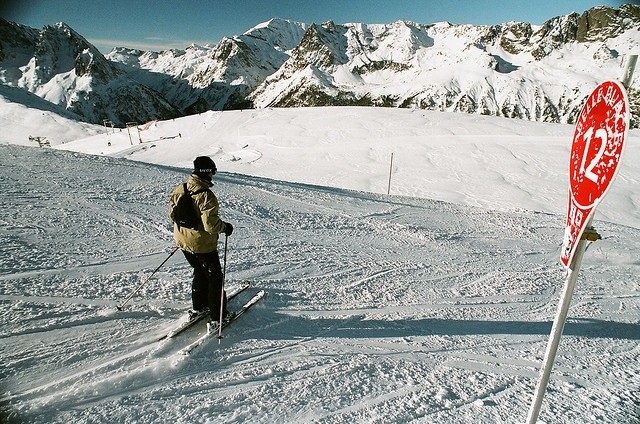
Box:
[170,182,209,231]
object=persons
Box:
[170,157,236,325]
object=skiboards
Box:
[159,281,265,355]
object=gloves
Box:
[223,221,233,236]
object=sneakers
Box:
[211,312,235,327]
[193,307,210,316]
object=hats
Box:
[194,156,216,176]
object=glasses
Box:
[195,168,217,175]
